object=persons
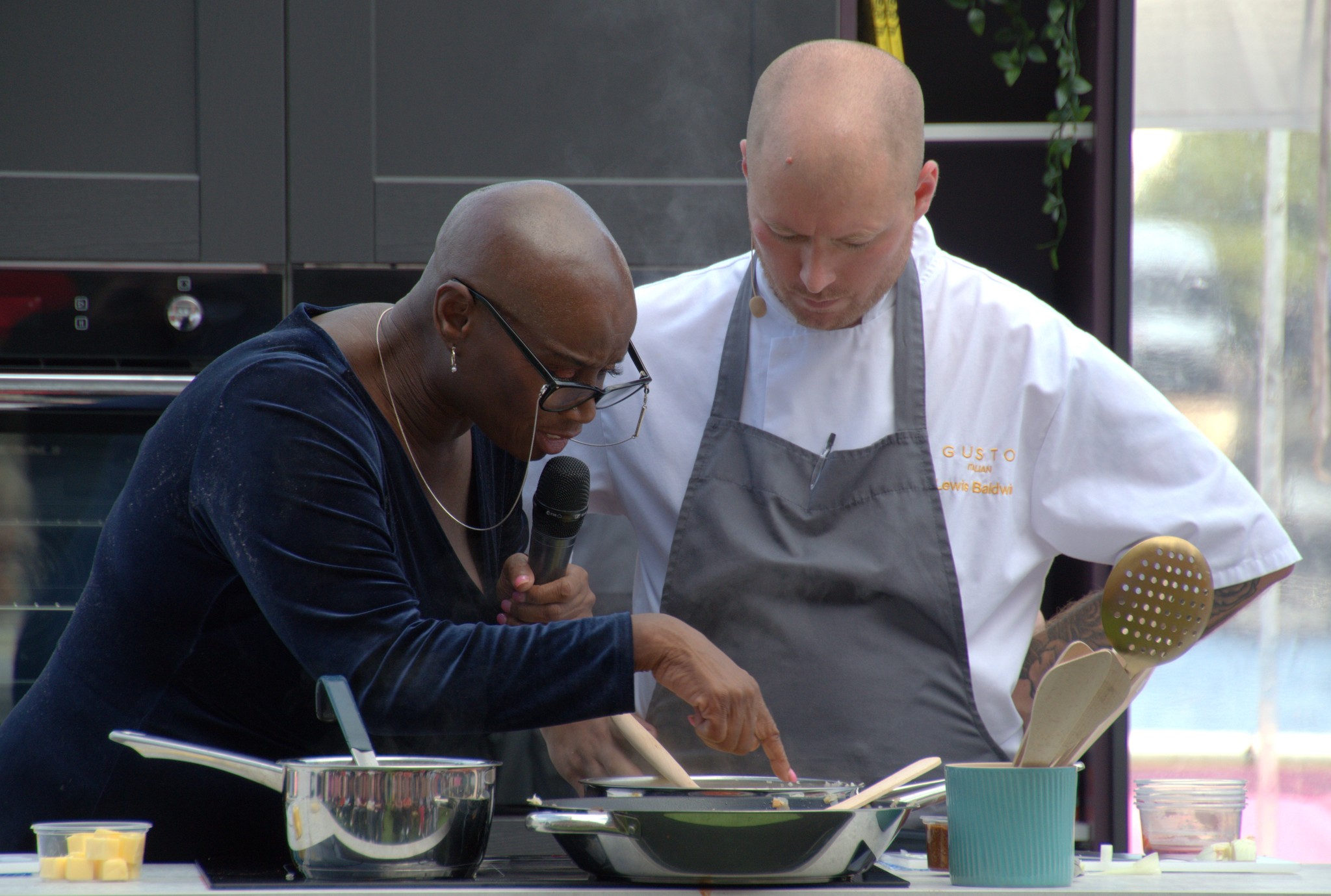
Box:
[0,178,796,883]
[523,39,1304,855]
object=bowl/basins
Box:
[30,820,152,880]
[1133,778,1248,862]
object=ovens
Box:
[0,256,440,737]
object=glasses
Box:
[452,278,652,411]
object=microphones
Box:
[528,455,590,586]
[749,231,771,318]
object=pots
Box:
[105,727,501,885]
[581,774,947,836]
[523,786,911,884]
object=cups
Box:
[947,762,1076,890]
[919,814,950,872]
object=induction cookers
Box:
[198,838,919,896]
